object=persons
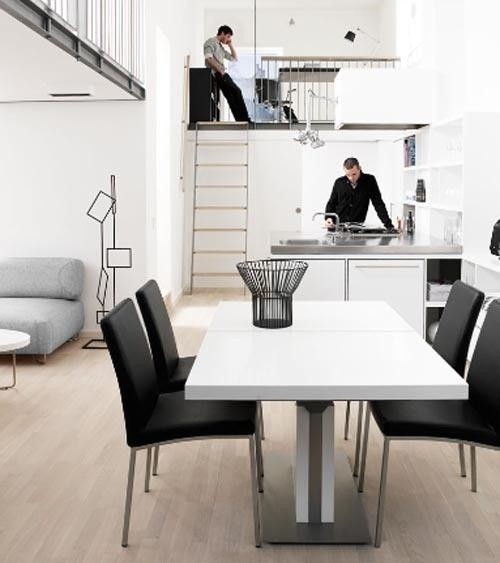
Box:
[324,157,398,235]
[203,25,253,125]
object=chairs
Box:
[255,76,294,121]
[344,277,487,477]
[135,277,266,478]
[356,297,500,548]
[100,298,264,548]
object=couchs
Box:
[1,255,85,365]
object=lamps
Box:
[343,26,383,66]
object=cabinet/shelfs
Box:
[189,67,221,122]
[460,259,500,312]
[291,259,424,339]
[401,119,463,249]
[425,258,463,344]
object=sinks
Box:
[284,238,323,247]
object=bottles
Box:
[407,210,413,234]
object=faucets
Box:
[311,211,340,234]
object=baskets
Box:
[236,259,309,329]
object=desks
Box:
[185,300,469,545]
